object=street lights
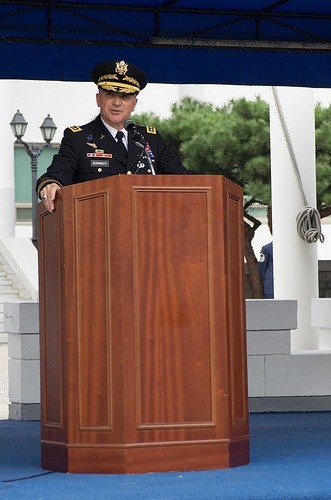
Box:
[9,109,57,247]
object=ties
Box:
[116,130,128,157]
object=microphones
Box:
[124,120,141,137]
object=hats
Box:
[91,61,148,94]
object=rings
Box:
[42,195,47,199]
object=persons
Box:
[258,240,273,299]
[35,60,209,214]
